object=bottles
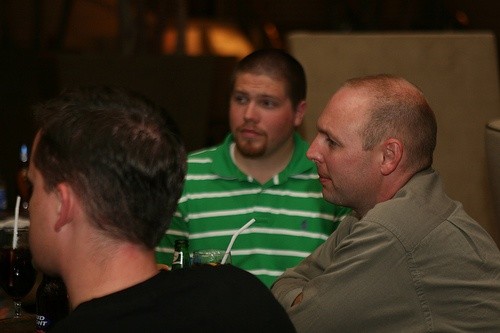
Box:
[170,238,191,273]
[15,143,33,216]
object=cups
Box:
[192,247,231,269]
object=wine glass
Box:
[1,225,37,319]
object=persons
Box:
[153,46,353,295]
[268,74,500,333]
[27,89,297,333]
